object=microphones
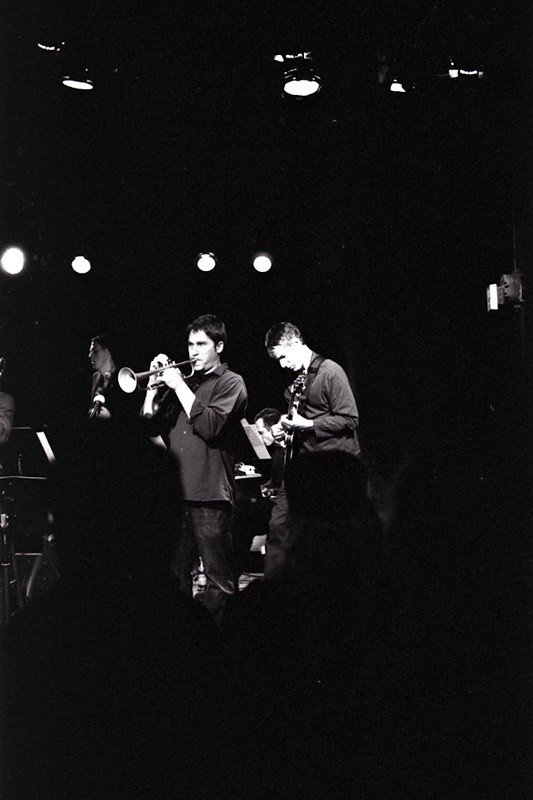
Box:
[89,395,105,417]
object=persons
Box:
[0,443,533,800]
[88,335,132,420]
[253,409,285,499]
[139,314,248,603]
[265,321,361,455]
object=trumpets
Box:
[117,356,198,394]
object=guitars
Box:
[279,373,306,468]
